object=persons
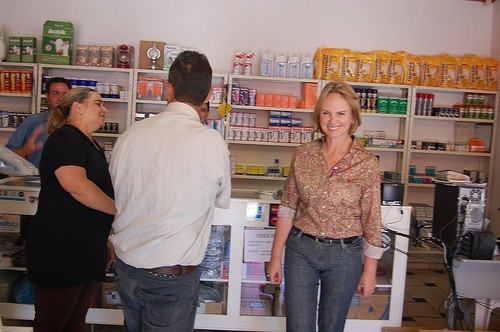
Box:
[27,87,116,332]
[108,50,231,332]
[267,81,390,332]
[5,77,72,304]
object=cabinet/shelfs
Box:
[0,61,500,271]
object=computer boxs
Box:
[432,182,486,252]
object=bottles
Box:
[415,92,494,120]
[354,87,378,112]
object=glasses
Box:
[48,90,59,95]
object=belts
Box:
[145,265,197,276]
[293,225,358,245]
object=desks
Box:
[426,240,500,332]
